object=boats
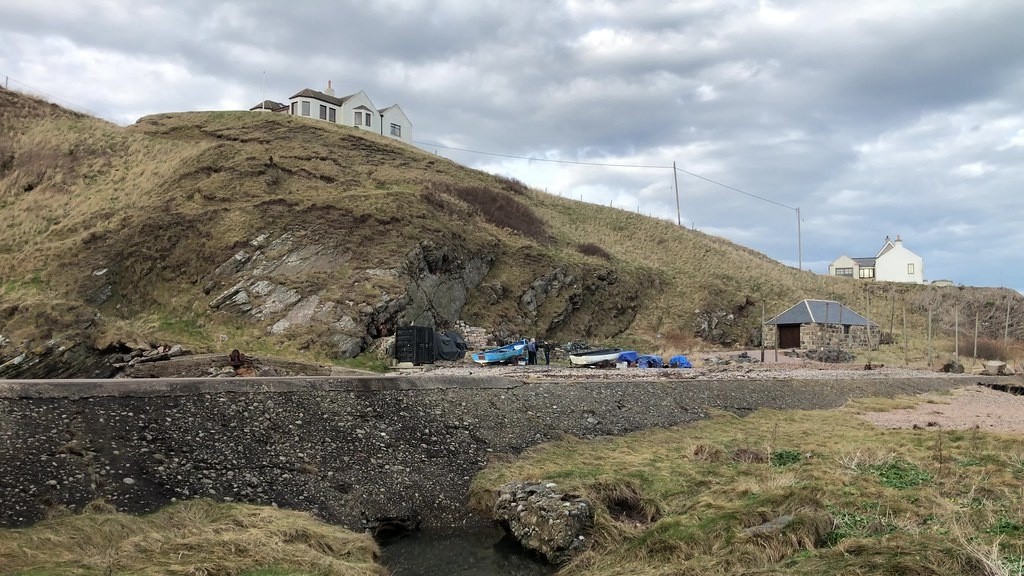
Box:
[470,338,529,367]
[569,347,625,367]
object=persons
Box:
[543,342,550,365]
[527,338,538,365]
